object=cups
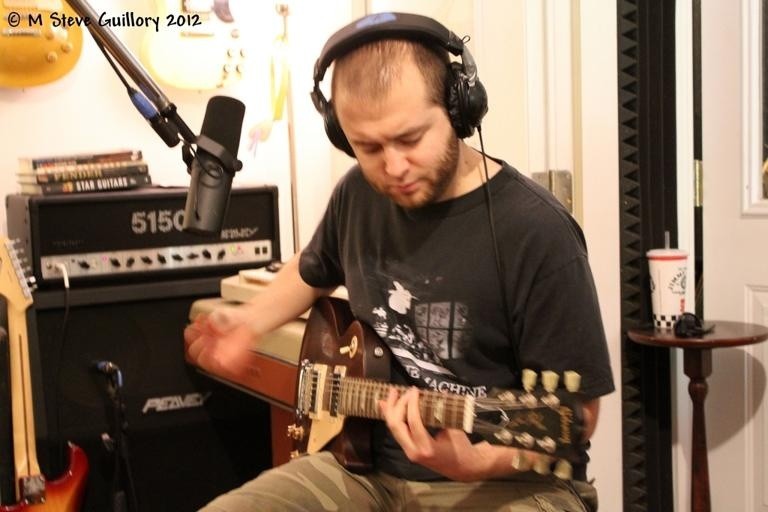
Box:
[645,248,690,332]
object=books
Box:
[11,148,156,199]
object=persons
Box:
[180,8,615,511]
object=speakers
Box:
[26,277,275,512]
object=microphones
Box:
[91,357,118,376]
[181,95,247,237]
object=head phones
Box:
[310,12,488,158]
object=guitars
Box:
[0,236,88,512]
[287,295,591,481]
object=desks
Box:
[627,321,768,512]
